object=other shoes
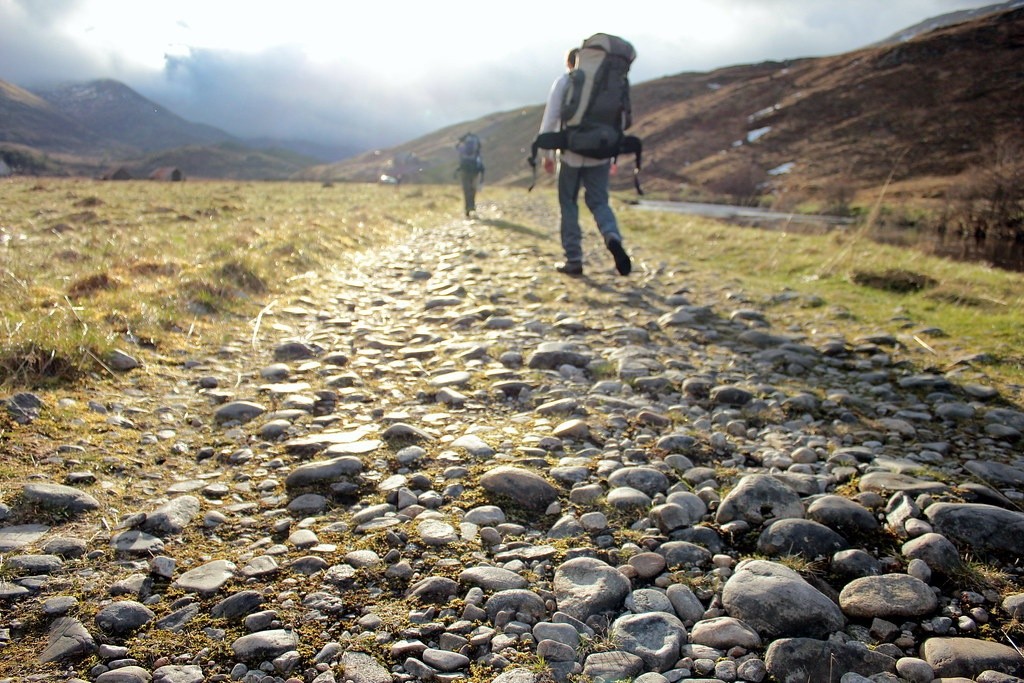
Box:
[557,261,583,275]
[603,232,631,275]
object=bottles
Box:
[476,156,481,170]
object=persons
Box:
[539,48,632,276]
[459,137,484,216]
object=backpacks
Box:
[459,132,482,174]
[560,32,637,159]
